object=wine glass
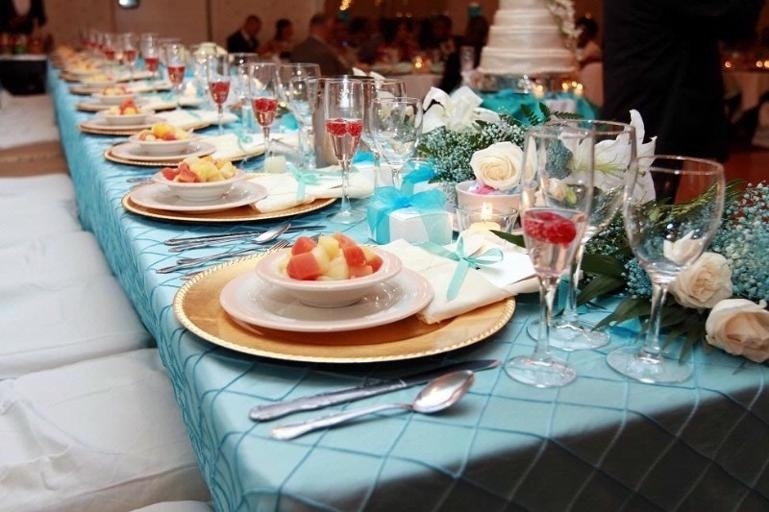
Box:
[245,61,283,159]
[370,97,423,187]
[528,121,638,351]
[356,79,408,167]
[285,75,319,158]
[324,78,368,224]
[607,154,726,386]
[506,127,597,388]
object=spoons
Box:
[261,368,476,441]
[170,221,292,252]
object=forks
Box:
[155,238,290,274]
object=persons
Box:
[438,17,489,96]
[0,0,47,37]
[225,14,262,54]
[333,12,456,64]
[256,18,299,59]
[575,16,602,68]
[289,12,369,76]
[600,0,765,210]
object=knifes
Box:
[248,356,503,422]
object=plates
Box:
[175,247,516,364]
[105,128,266,167]
[50,27,426,115]
[121,169,341,222]
[80,107,216,135]
